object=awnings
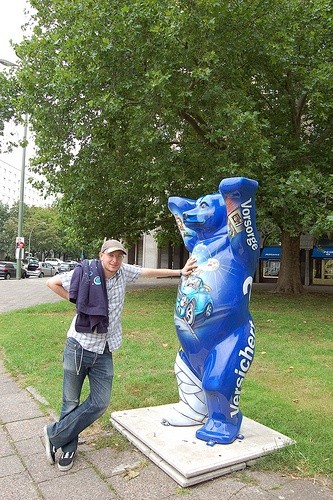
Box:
[261,246,282,258]
[311,246,333,258]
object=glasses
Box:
[102,252,124,259]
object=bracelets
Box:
[179,269,182,276]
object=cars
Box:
[23,256,59,278]
[58,262,75,273]
[0,261,27,280]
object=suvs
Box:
[45,257,65,266]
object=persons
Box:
[43,238,199,472]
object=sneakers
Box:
[44,425,57,465]
[58,451,76,472]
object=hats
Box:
[101,240,127,256]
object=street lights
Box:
[0,58,28,279]
